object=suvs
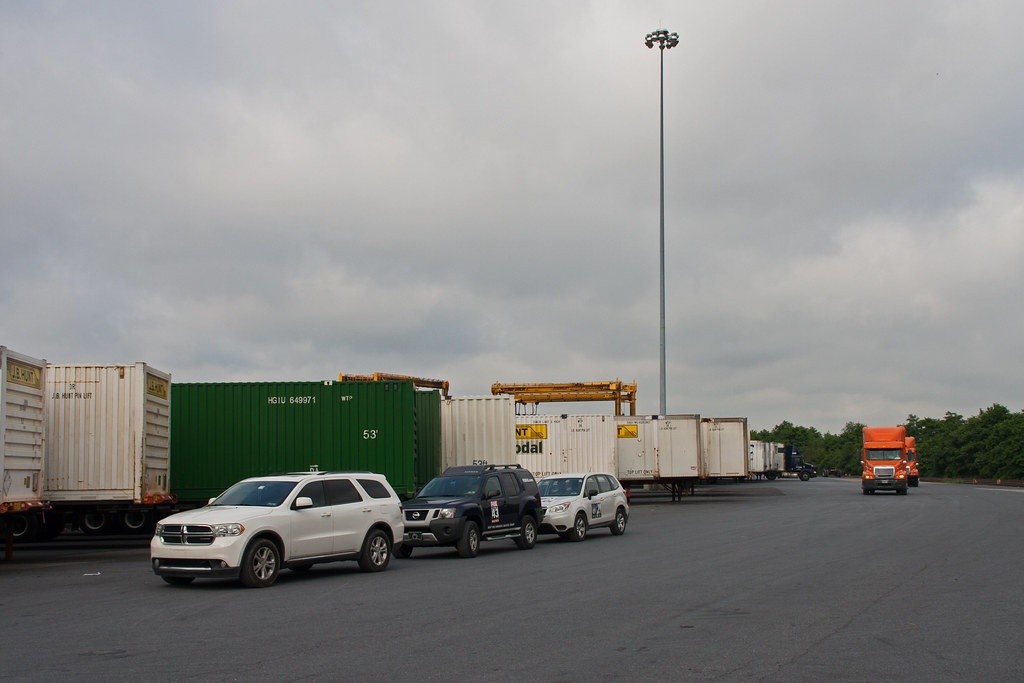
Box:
[151,465,405,589]
[537,473,630,541]
[391,464,543,560]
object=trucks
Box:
[765,444,817,481]
[859,426,907,495]
[905,437,919,487]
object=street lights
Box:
[646,28,678,417]
